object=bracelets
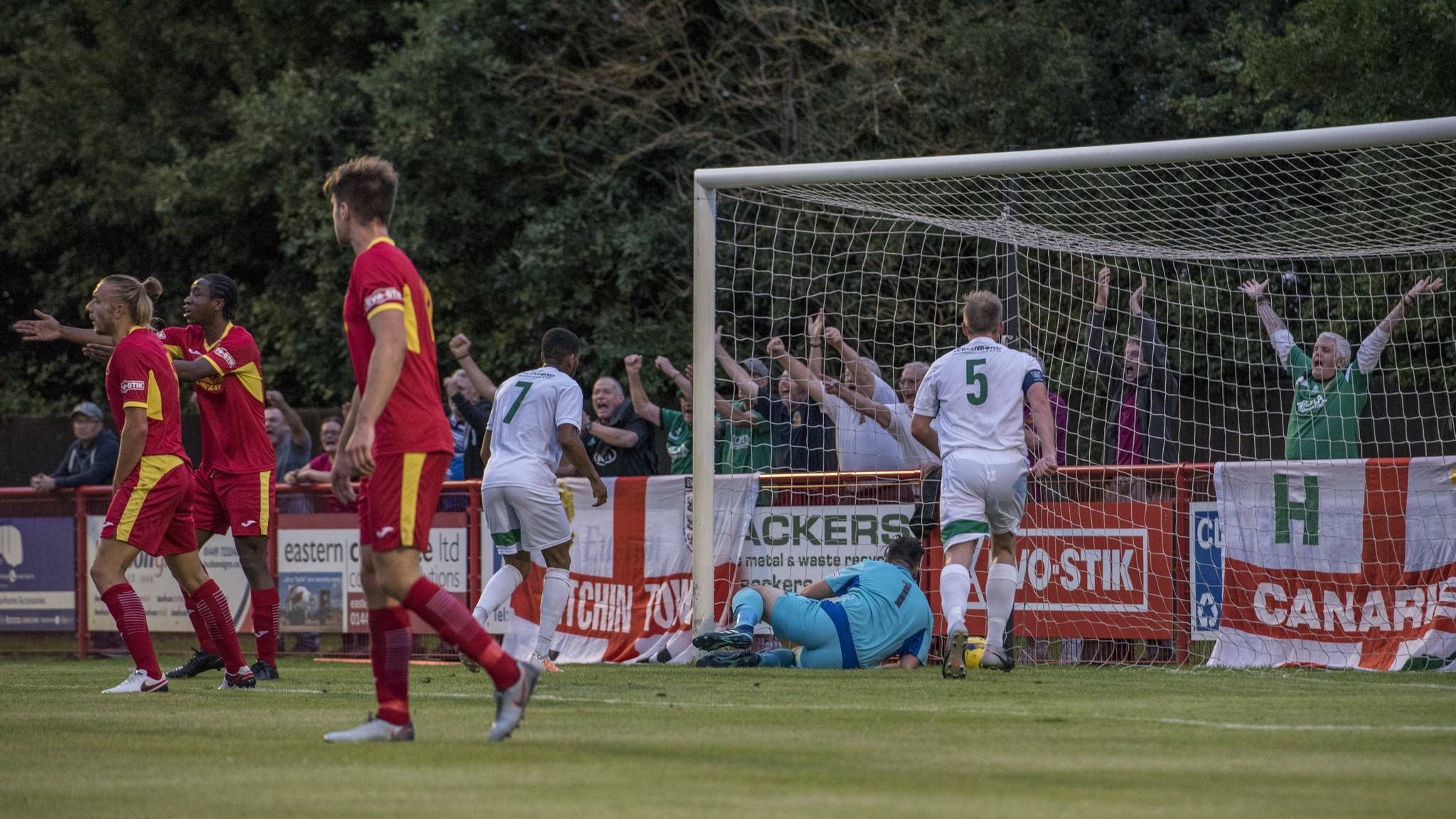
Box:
[809,341,822,348]
[1399,292,1415,308]
[671,371,681,380]
[1252,293,1271,308]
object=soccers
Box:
[961,636,988,668]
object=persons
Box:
[84,273,257,696]
[1087,267,1182,664]
[1237,275,1444,459]
[29,401,119,490]
[457,326,610,676]
[322,152,541,745]
[1017,347,1084,665]
[12,270,281,682]
[260,387,312,514]
[556,308,942,507]
[688,536,934,671]
[910,290,1057,677]
[286,334,498,512]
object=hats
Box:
[72,402,103,422]
[677,390,685,399]
[738,358,768,377]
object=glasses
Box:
[320,429,339,437]
[73,418,100,425]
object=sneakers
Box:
[979,644,1015,673]
[101,667,169,694]
[692,627,754,652]
[530,650,565,673]
[218,665,256,689]
[322,710,415,743]
[696,649,762,668]
[489,659,541,742]
[942,620,969,679]
[459,652,480,673]
[250,658,279,680]
[165,647,225,678]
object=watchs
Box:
[585,421,593,432]
